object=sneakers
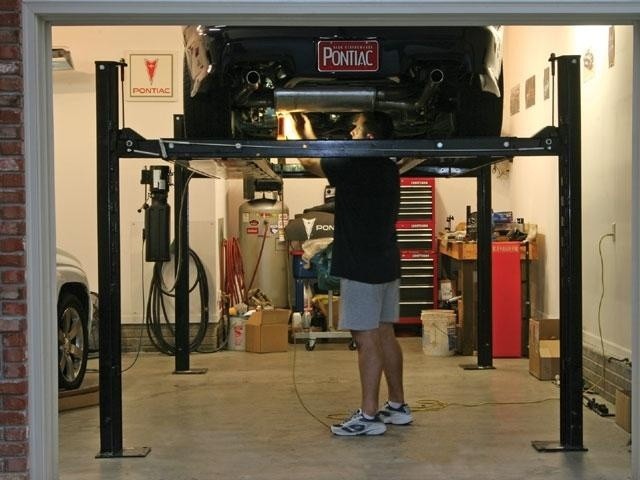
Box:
[331,406,387,437]
[376,399,413,426]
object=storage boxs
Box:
[612,388,632,430]
[527,316,562,381]
[227,303,290,356]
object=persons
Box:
[273,105,415,440]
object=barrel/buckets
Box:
[420,309,457,358]
[227,316,250,352]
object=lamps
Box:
[48,48,75,73]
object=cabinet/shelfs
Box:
[94,55,587,459]
[393,178,443,326]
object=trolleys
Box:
[291,289,358,350]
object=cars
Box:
[55,247,94,392]
[176,24,504,184]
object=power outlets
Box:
[610,223,617,242]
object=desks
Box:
[441,238,539,358]
